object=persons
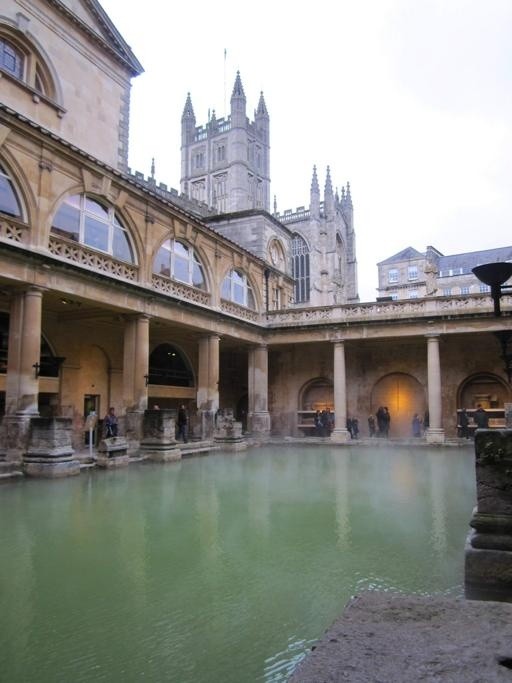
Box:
[347,416,359,439]
[214,408,223,429]
[100,407,118,457]
[459,408,471,441]
[314,408,334,437]
[375,406,391,439]
[153,403,160,409]
[367,414,376,437]
[175,404,190,444]
[412,414,423,437]
[422,411,429,430]
[473,404,489,429]
